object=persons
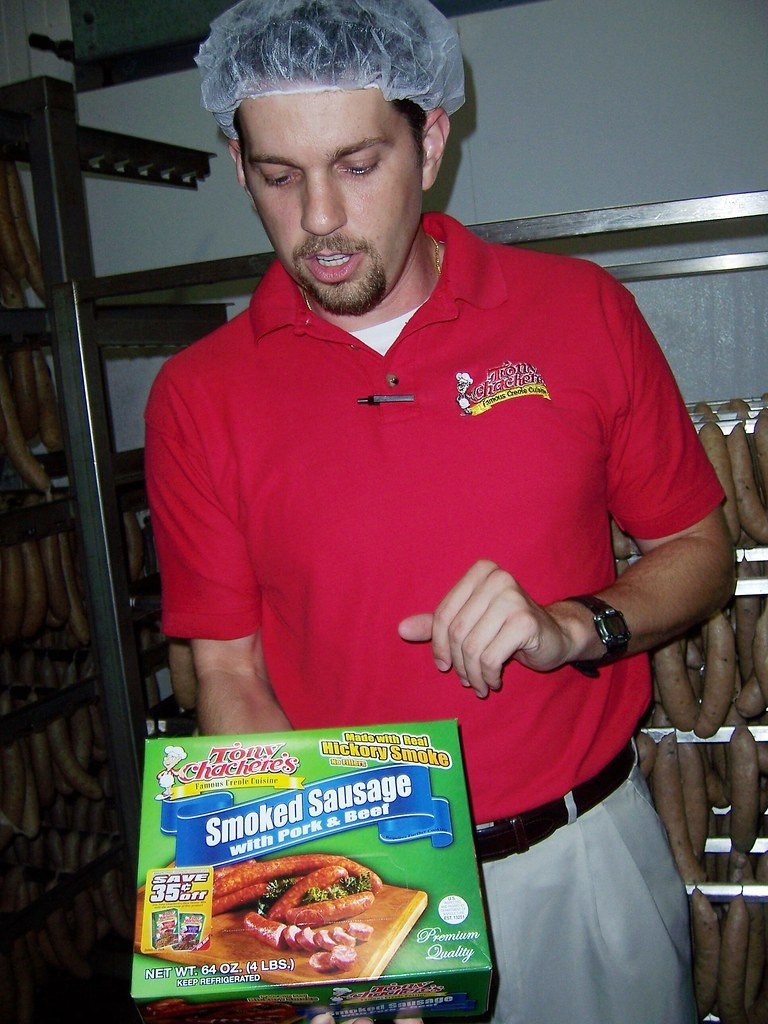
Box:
[144,2,743,1024]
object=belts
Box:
[477,738,633,864]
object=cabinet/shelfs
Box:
[0,72,768,1024]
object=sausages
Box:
[0,157,132,1024]
[208,851,383,974]
[608,395,768,1023]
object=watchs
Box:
[564,595,633,681]
